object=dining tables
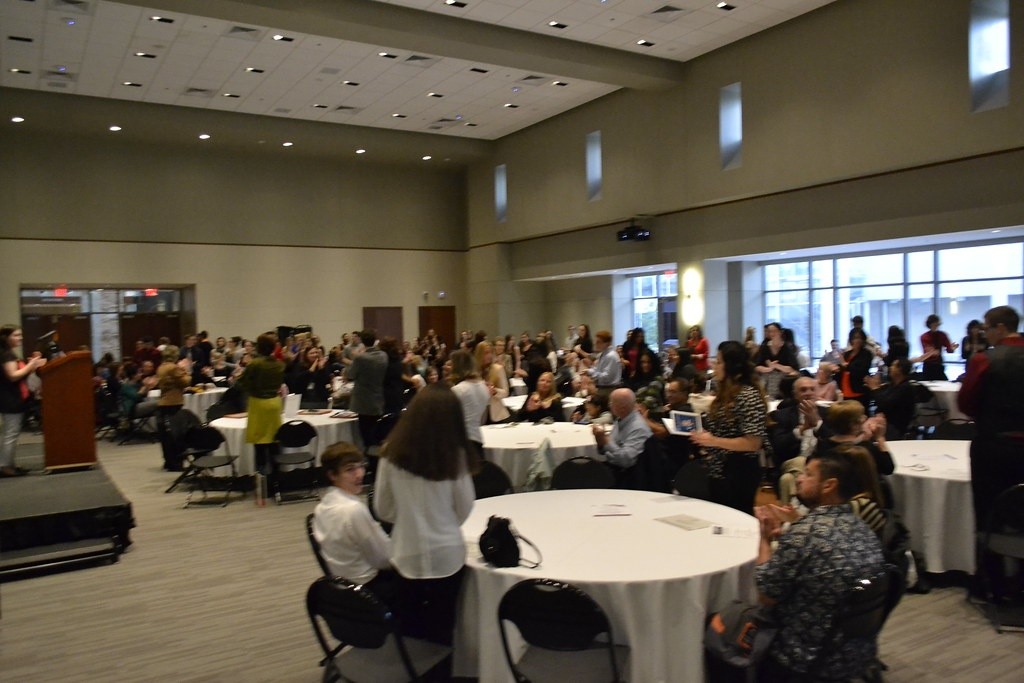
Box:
[905,377,978,437]
[754,398,838,475]
[862,434,986,582]
[472,422,614,495]
[437,487,762,682]
[131,382,230,438]
[495,390,592,423]
[205,405,365,492]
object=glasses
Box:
[980,325,994,335]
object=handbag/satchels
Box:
[480,516,542,568]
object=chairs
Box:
[176,423,247,509]
[546,455,615,493]
[114,396,160,446]
[925,417,986,446]
[669,460,712,503]
[294,573,436,683]
[305,510,401,632]
[88,389,127,442]
[493,576,630,683]
[967,484,1023,634]
[362,413,401,476]
[264,418,322,505]
[718,563,907,681]
[464,459,516,497]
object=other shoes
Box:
[0,467,32,477]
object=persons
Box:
[956,308,1023,604]
[768,445,896,545]
[373,381,475,681]
[313,442,421,640]
[1,307,1024,515]
[696,450,890,681]
